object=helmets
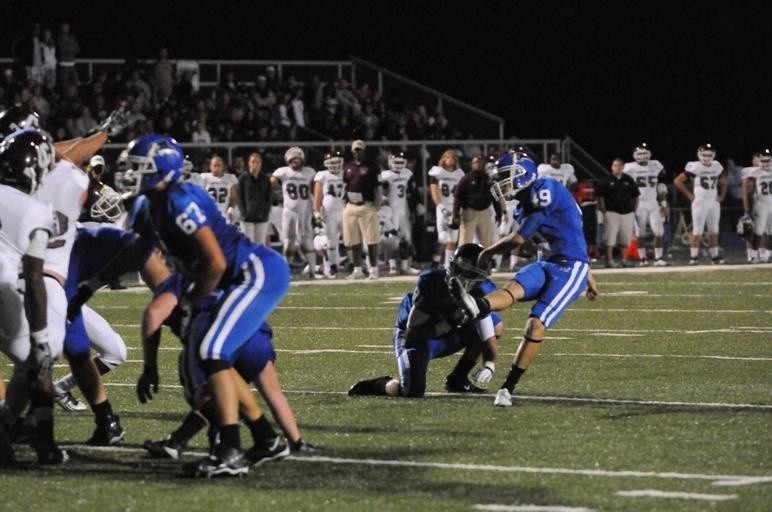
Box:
[445,243,493,291]
[488,151,538,202]
[79,176,125,222]
[113,134,185,198]
[757,149,772,168]
[0,108,56,195]
[388,150,408,172]
[285,147,305,164]
[697,144,716,166]
[323,152,344,174]
[633,143,651,166]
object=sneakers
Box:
[37,449,73,467]
[605,258,670,267]
[85,415,126,447]
[494,388,513,407]
[313,263,421,279]
[446,375,488,393]
[196,432,324,478]
[448,276,481,320]
[714,257,726,264]
[145,437,188,459]
[50,382,88,410]
[689,257,698,264]
[348,376,390,397]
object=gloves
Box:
[21,340,51,388]
[448,218,461,230]
[66,284,94,324]
[471,366,494,385]
[311,215,325,233]
[137,364,159,403]
[80,102,146,139]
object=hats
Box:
[352,140,365,152]
[90,155,105,168]
